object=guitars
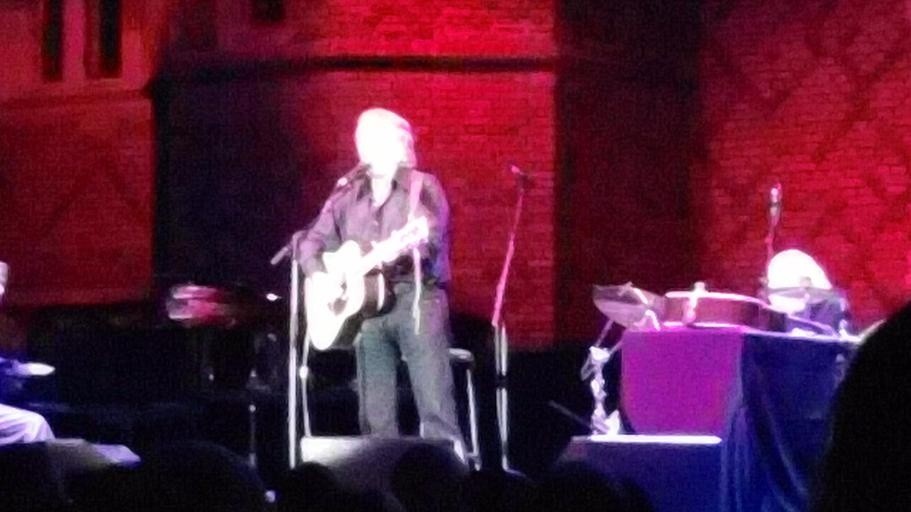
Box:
[303,216,432,351]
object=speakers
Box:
[0,438,141,512]
[300,435,469,512]
[537,437,721,512]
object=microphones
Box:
[770,185,780,226]
[507,163,531,181]
[336,160,371,187]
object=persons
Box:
[300,108,468,466]
[764,247,857,333]
[0,260,54,444]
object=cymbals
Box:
[768,291,847,300]
[593,286,666,331]
